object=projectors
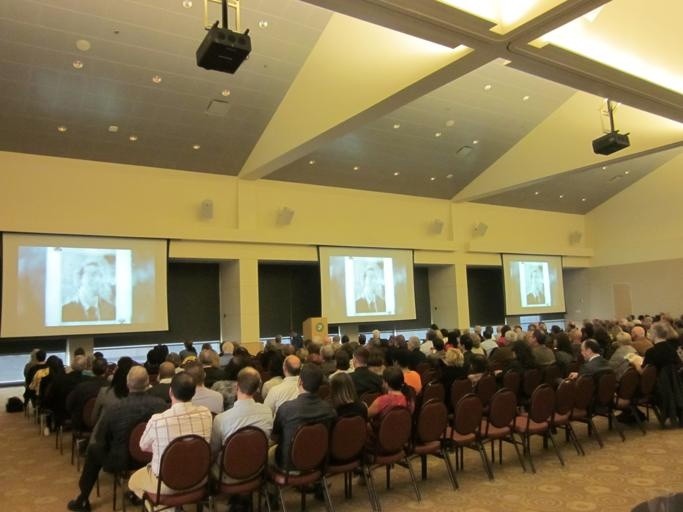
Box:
[197,21,252,74]
[593,130,631,154]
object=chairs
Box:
[20,333,683,511]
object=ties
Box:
[370,299,376,312]
[86,306,99,321]
[535,294,539,304]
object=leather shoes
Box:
[67,499,91,512]
[125,491,147,506]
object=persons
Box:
[525,269,545,304]
[61,257,115,321]
[356,264,385,312]
